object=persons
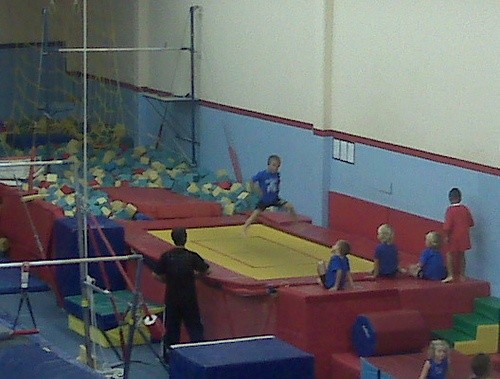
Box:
[315,240,364,292]
[400,229,449,283]
[441,187,476,283]
[151,225,211,363]
[420,340,451,378]
[240,155,296,235]
[360,223,399,278]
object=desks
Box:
[56,215,125,296]
[169,336,316,379]
